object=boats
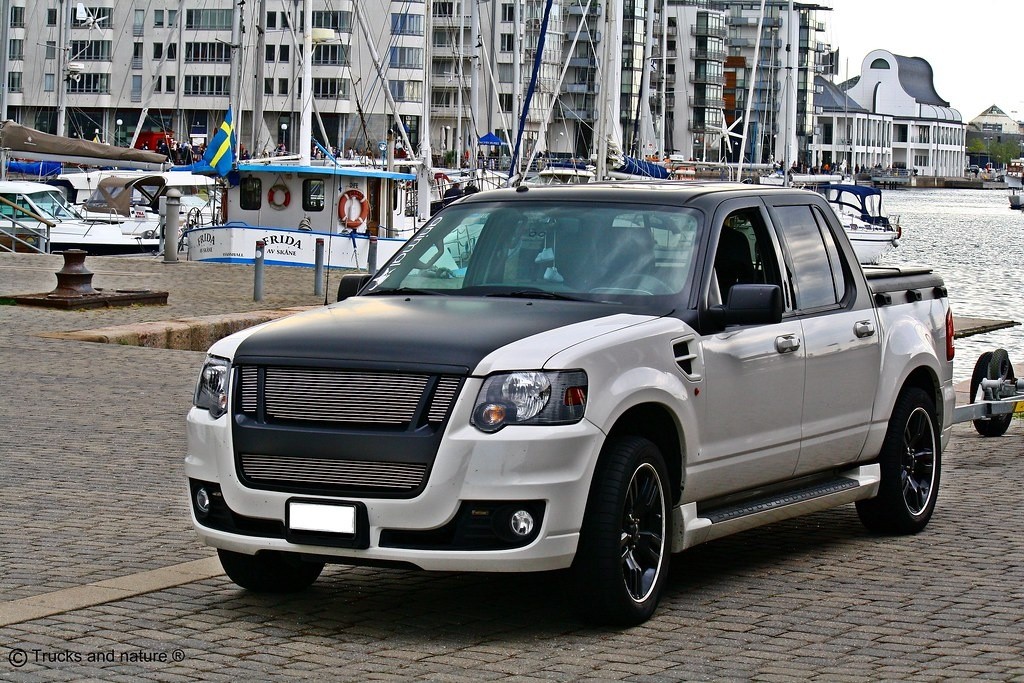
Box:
[789,182,902,266]
[4,1,718,252]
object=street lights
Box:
[694,132,707,164]
[440,124,450,160]
[115,118,124,148]
[279,122,289,152]
[555,129,564,159]
[731,140,738,161]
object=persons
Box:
[444,180,463,206]
[311,145,408,160]
[240,142,250,159]
[760,159,896,175]
[974,164,990,178]
[476,148,555,171]
[140,139,208,164]
[277,144,286,152]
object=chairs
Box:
[534,226,655,291]
[713,226,753,307]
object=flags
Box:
[203,105,236,178]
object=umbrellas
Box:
[477,131,506,154]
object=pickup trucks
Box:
[181,180,957,624]
